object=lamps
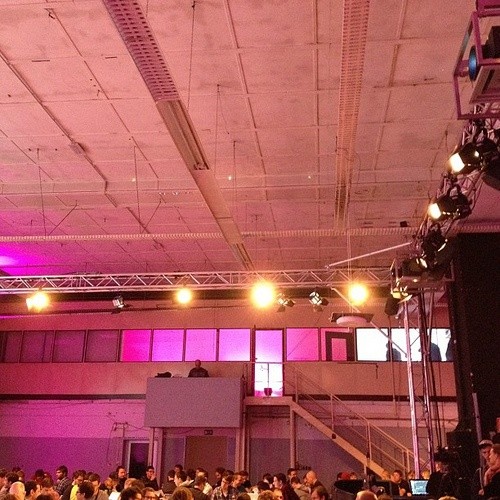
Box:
[428,184,473,220]
[416,223,447,269]
[448,136,498,174]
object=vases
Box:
[264,388,272,396]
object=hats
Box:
[341,472,351,480]
[35,469,44,477]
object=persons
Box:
[188,360,209,377]
[386,343,401,361]
[418,336,442,361]
[0,439,500,500]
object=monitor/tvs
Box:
[409,479,430,496]
